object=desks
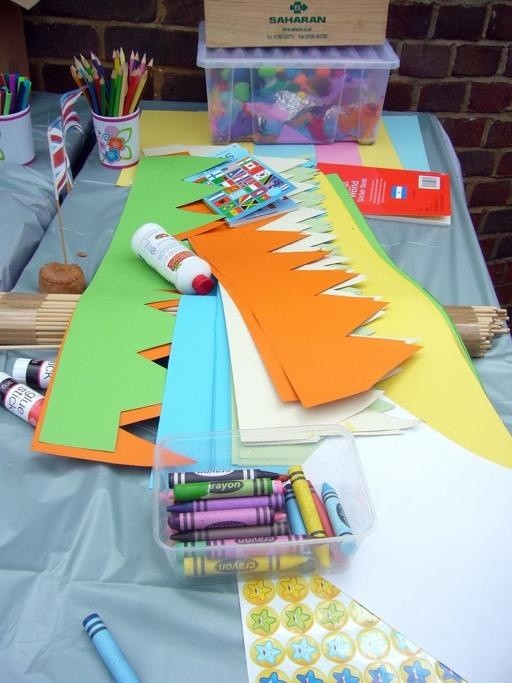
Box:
[0,89,96,293]
[0,97,511,683]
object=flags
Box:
[49,85,89,195]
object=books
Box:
[316,163,451,226]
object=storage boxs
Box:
[196,18,401,146]
[152,421,375,580]
[202,1,389,48]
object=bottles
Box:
[131,222,215,295]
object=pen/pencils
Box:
[70,47,154,117]
[0,72,31,116]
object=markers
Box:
[166,467,357,577]
[0,371,46,427]
[82,613,140,683]
[13,358,55,390]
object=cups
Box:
[0,103,36,165]
[91,106,141,169]
